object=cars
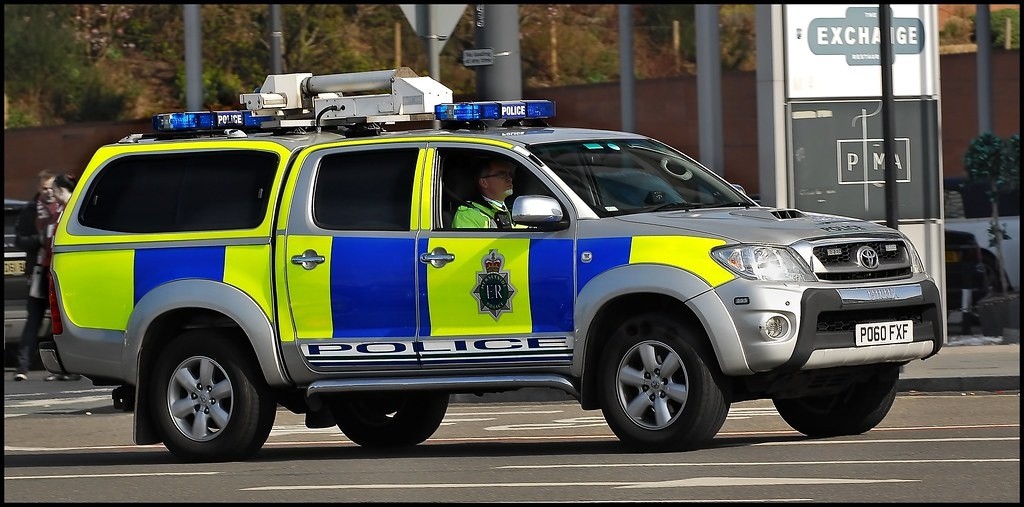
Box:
[5,199,52,345]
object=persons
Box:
[450,155,537,228]
[11,169,59,381]
[43,171,80,380]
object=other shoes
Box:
[45,375,57,381]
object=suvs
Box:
[37,67,943,462]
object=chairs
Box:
[440,172,480,230]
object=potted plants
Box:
[962,132,1021,338]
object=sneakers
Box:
[14,372,27,380]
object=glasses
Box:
[481,171,515,179]
